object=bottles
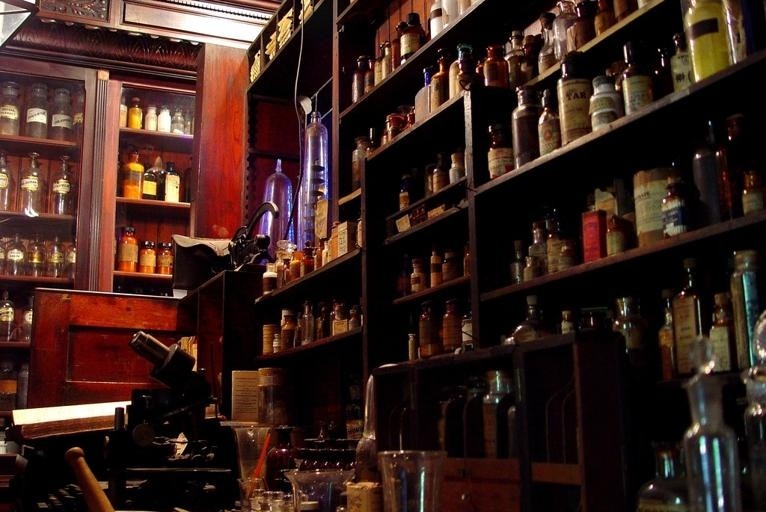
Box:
[27,234,48,279]
[365,0,766,509]
[255,219,362,357]
[0,80,22,136]
[50,87,73,142]
[0,234,7,276]
[183,158,193,202]
[144,104,158,130]
[158,106,172,132]
[51,154,72,216]
[144,156,164,199]
[119,226,138,272]
[140,240,157,274]
[120,89,128,128]
[114,236,118,270]
[185,109,194,135]
[172,105,185,133]
[0,149,12,212]
[24,83,50,140]
[21,151,41,218]
[47,234,66,277]
[117,152,123,196]
[7,232,28,278]
[2,287,34,414]
[122,152,143,199]
[128,97,143,129]
[164,161,182,202]
[67,237,77,278]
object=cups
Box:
[234,422,271,480]
[282,466,353,511]
[378,447,448,512]
[344,481,379,512]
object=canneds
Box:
[460,313,473,348]
[263,324,276,353]
[137,240,157,273]
[116,227,139,272]
